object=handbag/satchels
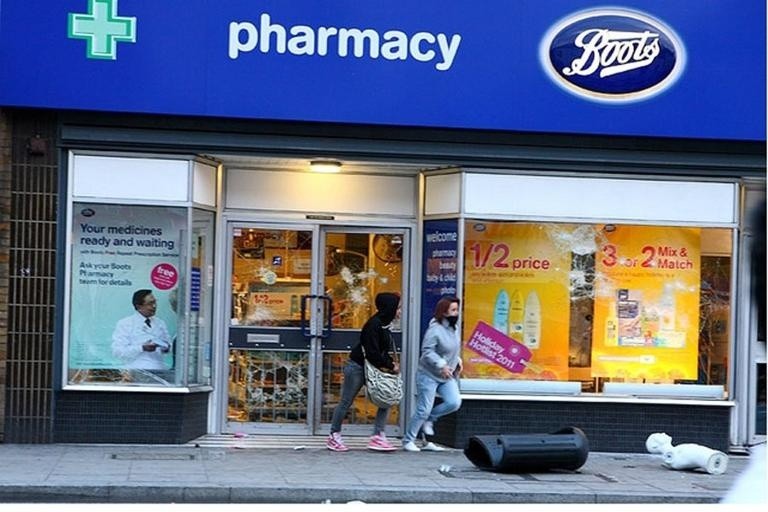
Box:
[363,358,407,408]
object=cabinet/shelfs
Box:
[244,359,361,423]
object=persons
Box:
[402,296,463,452]
[716,193,766,505]
[111,289,171,370]
[646,431,729,475]
[325,292,402,452]
[340,311,353,330]
[248,308,308,421]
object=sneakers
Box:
[422,420,435,436]
[325,432,350,453]
[403,441,421,452]
[367,431,396,451]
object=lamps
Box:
[310,160,342,174]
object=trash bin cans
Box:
[465,425,588,473]
